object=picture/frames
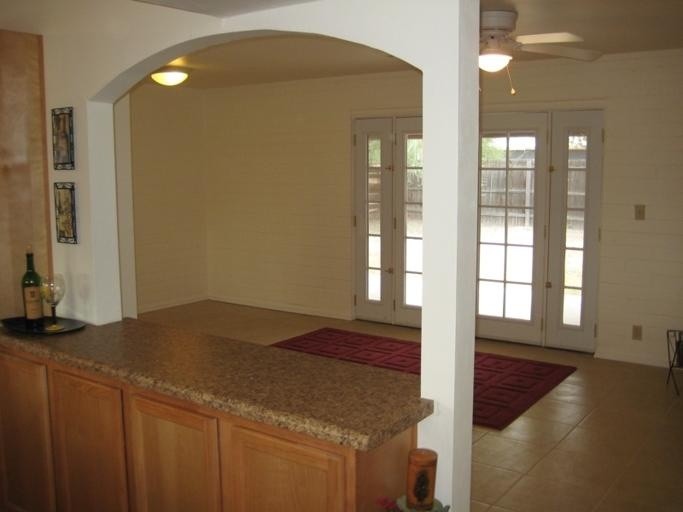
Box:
[51,105,77,246]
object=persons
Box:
[58,191,73,237]
[53,118,69,164]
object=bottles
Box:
[21,245,45,325]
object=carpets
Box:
[265,326,577,433]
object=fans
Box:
[478,10,601,72]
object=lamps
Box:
[478,35,517,73]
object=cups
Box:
[39,272,67,331]
